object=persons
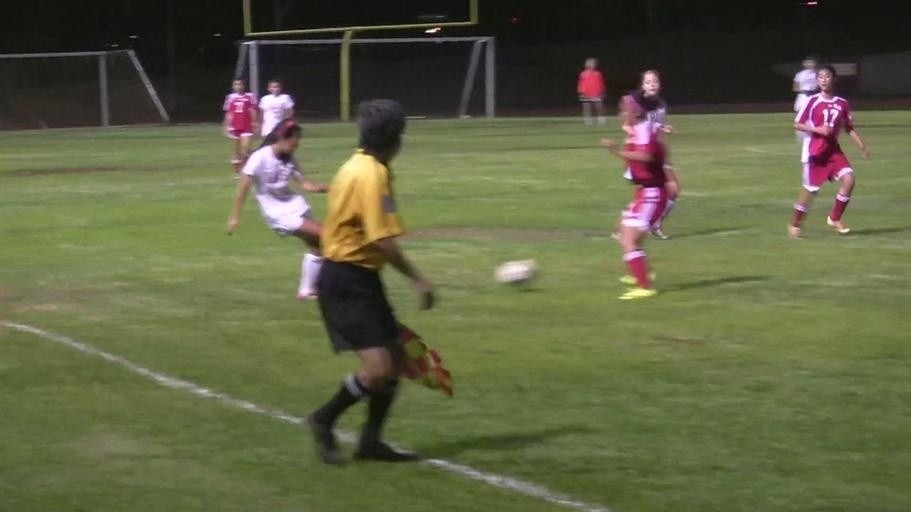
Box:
[258,80,297,145]
[792,56,821,144]
[225,117,335,300]
[222,78,260,178]
[576,56,608,127]
[623,69,680,239]
[598,90,667,301]
[306,96,435,464]
[788,67,869,241]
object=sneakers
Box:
[353,440,418,460]
[619,287,659,300]
[610,229,624,241]
[787,220,805,240]
[648,225,672,240]
[233,172,241,180]
[619,271,657,284]
[297,288,320,300]
[583,116,609,127]
[307,411,342,464]
[827,216,851,234]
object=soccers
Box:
[494,259,538,288]
